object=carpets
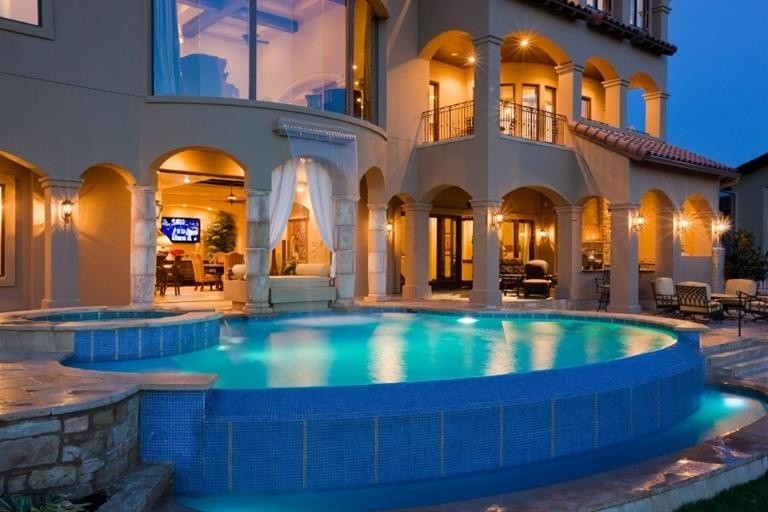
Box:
[701,337,768,388]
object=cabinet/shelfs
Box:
[595,263,768,336]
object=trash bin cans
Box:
[305,88,347,115]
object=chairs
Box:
[499,258,553,300]
[178,54,228,99]
[156,248,245,296]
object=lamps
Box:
[714,221,725,234]
[61,195,73,223]
[387,222,392,235]
[225,181,237,204]
[492,211,504,228]
[154,199,163,219]
[632,211,643,232]
[539,228,548,238]
[677,214,688,232]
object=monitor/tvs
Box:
[161,217,200,242]
